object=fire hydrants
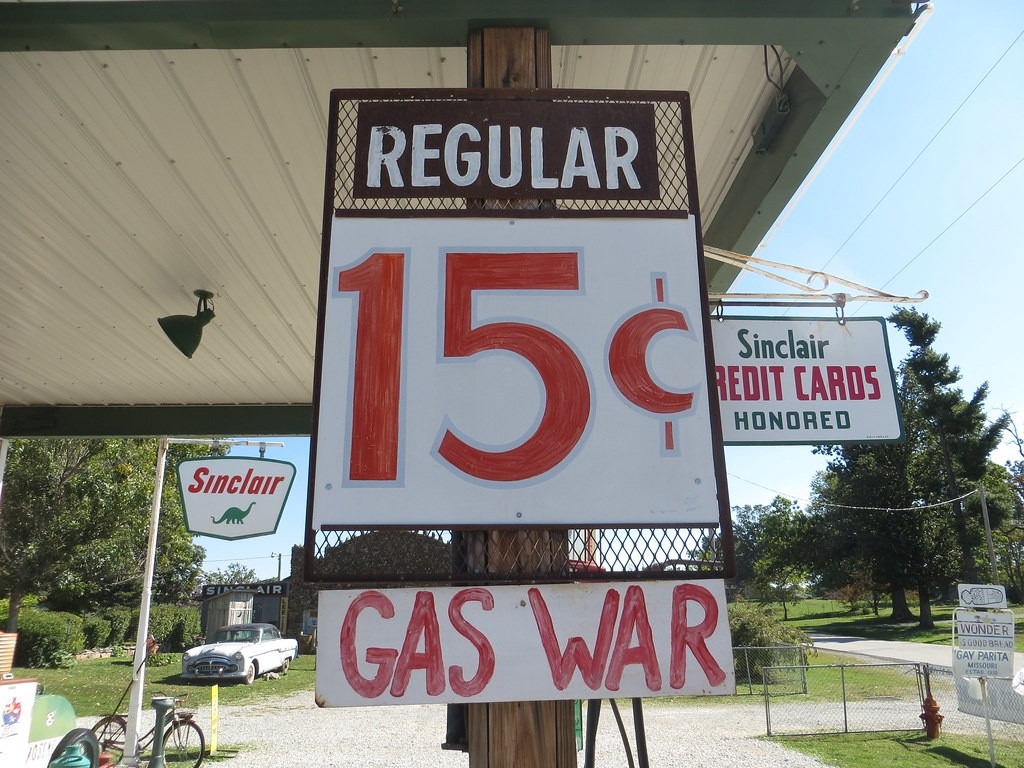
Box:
[918,696,946,739]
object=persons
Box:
[174,618,186,641]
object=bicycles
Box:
[88,690,206,768]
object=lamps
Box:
[157,289,216,359]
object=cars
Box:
[179,622,300,686]
[793,590,813,600]
